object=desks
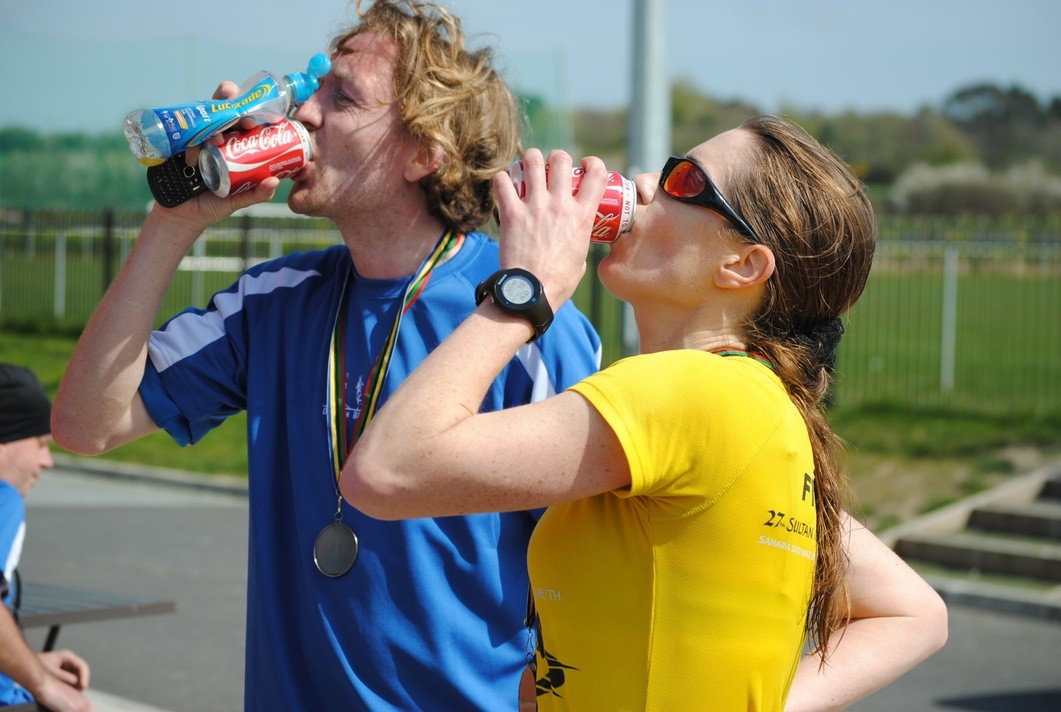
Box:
[15,583,177,654]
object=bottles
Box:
[124,52,332,166]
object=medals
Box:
[518,667,537,712]
[315,521,357,577]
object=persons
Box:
[51,0,603,712]
[342,114,950,712]
[0,362,90,712]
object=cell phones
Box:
[145,151,208,209]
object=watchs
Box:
[476,267,554,343]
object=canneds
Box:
[199,117,313,197]
[507,158,638,245]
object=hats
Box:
[0,359,54,445]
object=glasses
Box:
[657,152,760,247]
